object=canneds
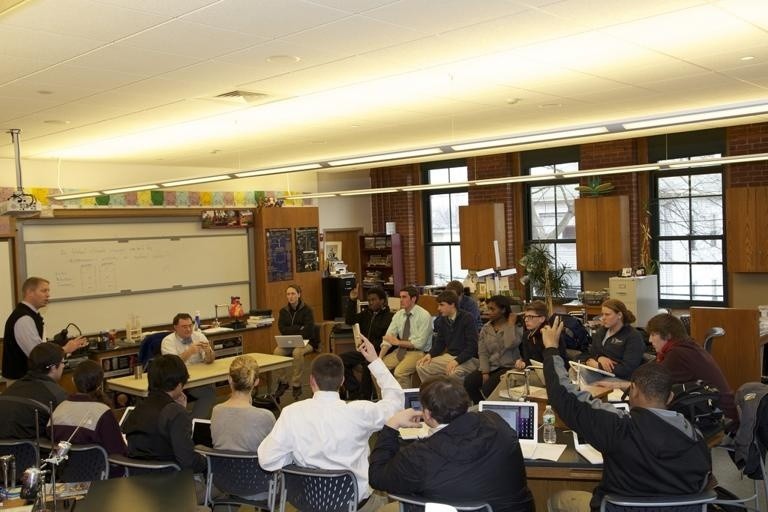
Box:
[56,441,72,458]
[23,467,40,490]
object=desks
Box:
[563,299,602,315]
[105,352,294,408]
[485,370,726,511]
[690,305,768,392]
[479,300,691,318]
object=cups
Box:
[134,366,143,380]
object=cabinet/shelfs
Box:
[94,329,249,410]
[727,187,768,273]
[459,204,506,271]
[574,195,630,271]
[358,232,404,298]
[254,207,322,354]
[609,275,659,329]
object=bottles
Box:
[543,406,557,443]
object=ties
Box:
[397,313,411,362]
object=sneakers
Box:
[292,387,302,396]
[275,383,290,397]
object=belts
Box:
[408,348,421,351]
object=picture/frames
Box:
[324,241,342,271]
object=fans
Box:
[475,240,518,295]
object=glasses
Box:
[522,315,541,320]
[46,360,69,369]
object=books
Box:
[362,235,394,288]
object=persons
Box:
[368,376,537,512]
[2,278,83,386]
[269,282,320,400]
[44,361,123,484]
[536,316,714,511]
[256,335,404,511]
[205,356,291,511]
[336,280,745,447]
[164,314,214,370]
[0,343,73,487]
[122,351,205,504]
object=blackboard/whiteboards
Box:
[16,216,257,344]
[0,238,15,341]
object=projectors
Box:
[0,193,41,219]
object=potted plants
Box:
[574,175,614,196]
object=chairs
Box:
[703,325,725,356]
[708,380,767,512]
[140,332,174,372]
[304,320,344,360]
[599,490,719,512]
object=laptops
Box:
[119,406,135,446]
[572,403,630,464]
[398,388,430,440]
[191,418,213,449]
[274,335,309,348]
[479,401,539,459]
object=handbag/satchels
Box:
[668,392,725,431]
[66,323,90,358]
[549,313,590,350]
